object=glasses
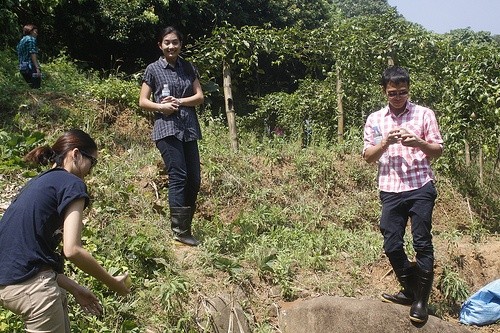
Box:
[386,89,408,98]
[80,152,98,167]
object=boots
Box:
[405,269,434,325]
[379,263,419,307]
[170,204,202,248]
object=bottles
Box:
[162,83,170,101]
[32,73,44,78]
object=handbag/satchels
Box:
[19,60,34,75]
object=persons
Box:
[139,26,204,246]
[0,130,130,333]
[362,65,444,322]
[17,24,42,89]
